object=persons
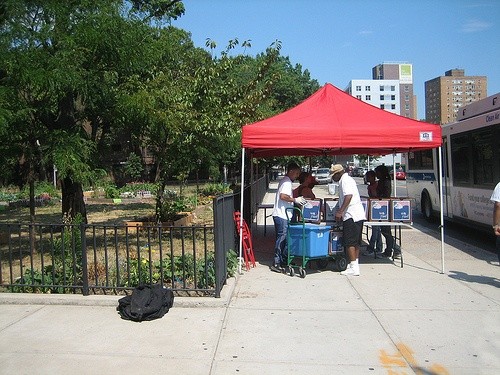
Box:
[328,164,367,276]
[291,171,319,222]
[271,162,307,273]
[490,182,500,235]
[362,165,404,261]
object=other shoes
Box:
[271,263,282,272]
[362,250,374,255]
[378,253,390,259]
[393,254,401,259]
[340,266,360,276]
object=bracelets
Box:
[493,224,499,229]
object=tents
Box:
[238,82,445,275]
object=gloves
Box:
[295,196,306,205]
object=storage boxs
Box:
[285,197,412,258]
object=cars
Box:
[389,169,406,180]
[303,163,376,177]
[313,169,334,185]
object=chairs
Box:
[233,210,256,270]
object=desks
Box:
[258,204,274,236]
[304,221,412,268]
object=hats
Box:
[329,164,343,176]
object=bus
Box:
[405,91,500,236]
